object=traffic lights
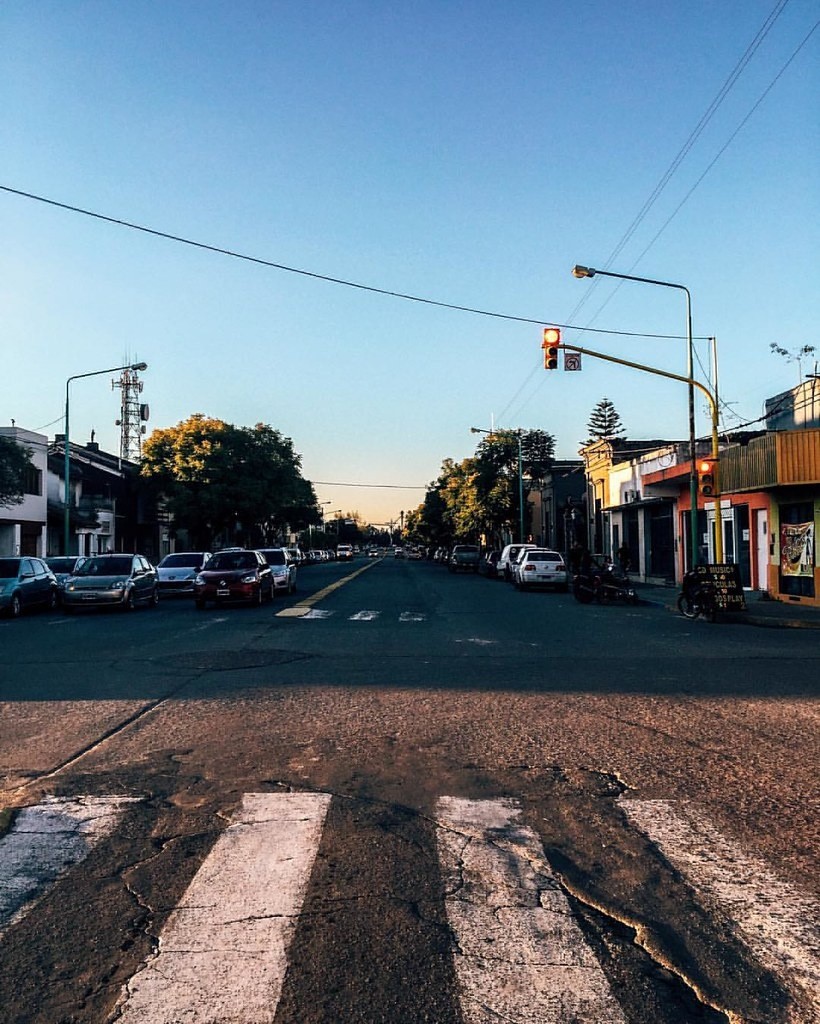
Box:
[544,328,560,369]
[698,459,717,497]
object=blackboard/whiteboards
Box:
[700,562,748,616]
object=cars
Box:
[281,543,361,566]
[394,547,403,559]
[254,548,298,595]
[154,551,219,598]
[0,556,58,618]
[368,549,378,557]
[41,555,97,593]
[60,552,160,612]
[405,541,569,593]
[193,550,275,610]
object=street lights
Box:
[471,426,523,543]
[63,362,148,556]
[569,263,700,570]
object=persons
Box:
[616,542,632,578]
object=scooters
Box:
[677,564,718,624]
[572,554,639,606]
